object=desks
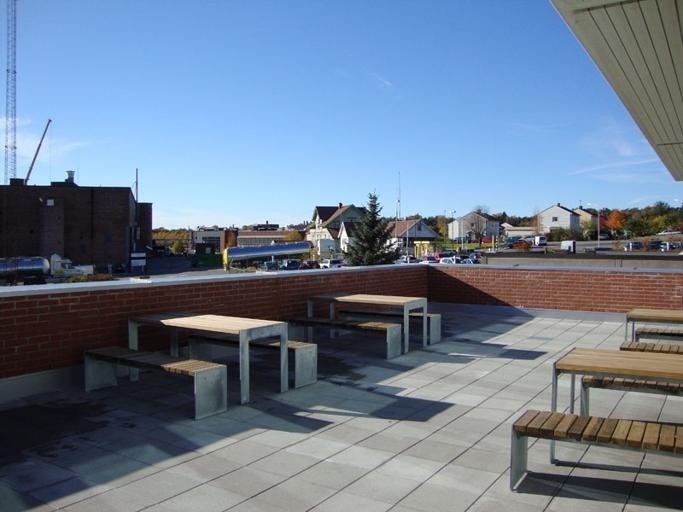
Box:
[126,312,289,406]
[624,309,683,340]
[306,294,427,353]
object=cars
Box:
[399,251,483,264]
[624,239,683,251]
[260,258,345,272]
[600,234,609,240]
[656,230,681,235]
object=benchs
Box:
[253,339,317,388]
[510,328,683,491]
[87,343,228,418]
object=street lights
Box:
[588,203,599,248]
[395,199,400,238]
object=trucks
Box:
[0,253,84,286]
[504,235,547,251]
